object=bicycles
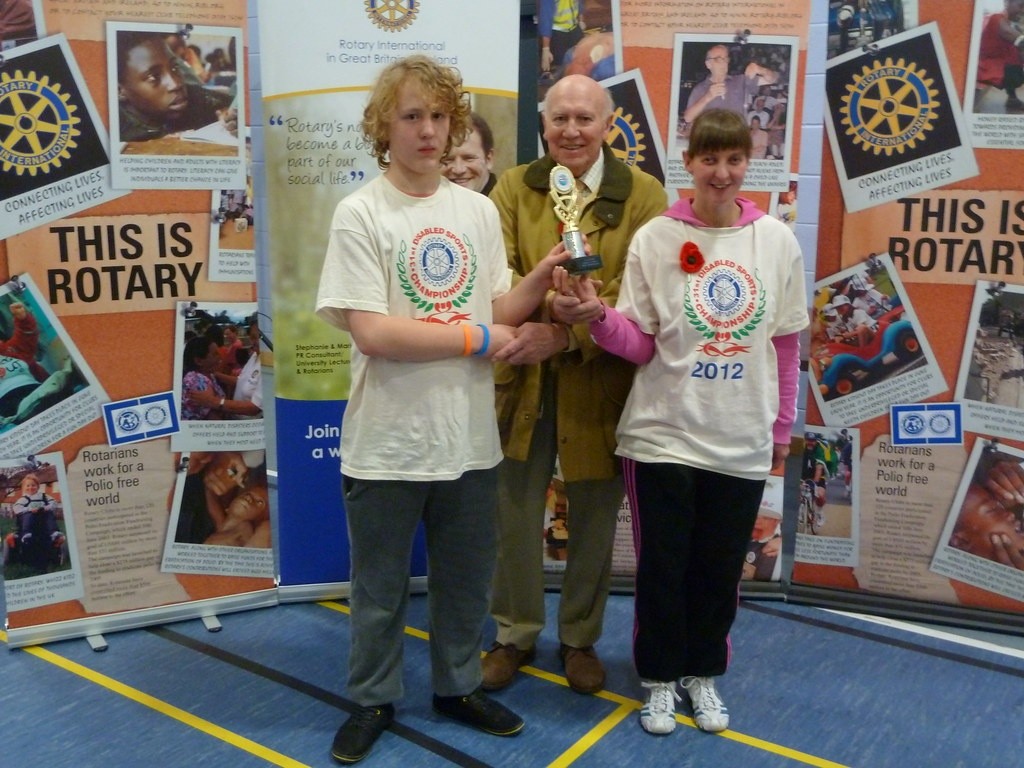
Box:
[799,478,819,536]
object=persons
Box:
[0,302,75,425]
[438,112,497,196]
[537,0,616,75]
[181,318,265,421]
[12,475,65,548]
[948,461,1024,572]
[544,483,558,538]
[801,429,853,528]
[175,451,272,549]
[684,44,791,159]
[117,31,239,142]
[814,272,892,348]
[742,496,782,581]
[314,55,669,762]
[552,106,809,734]
[977,0,1024,111]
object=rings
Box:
[570,290,574,294]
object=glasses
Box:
[708,56,731,63]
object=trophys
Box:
[549,165,604,274]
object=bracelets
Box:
[463,323,472,357]
[218,397,225,411]
[473,324,488,356]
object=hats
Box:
[850,277,875,291]
[822,295,851,318]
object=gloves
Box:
[542,46,554,72]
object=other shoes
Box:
[560,644,606,695]
[480,640,537,691]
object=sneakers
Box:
[331,703,396,763]
[679,676,729,732]
[434,685,526,736]
[640,679,683,735]
